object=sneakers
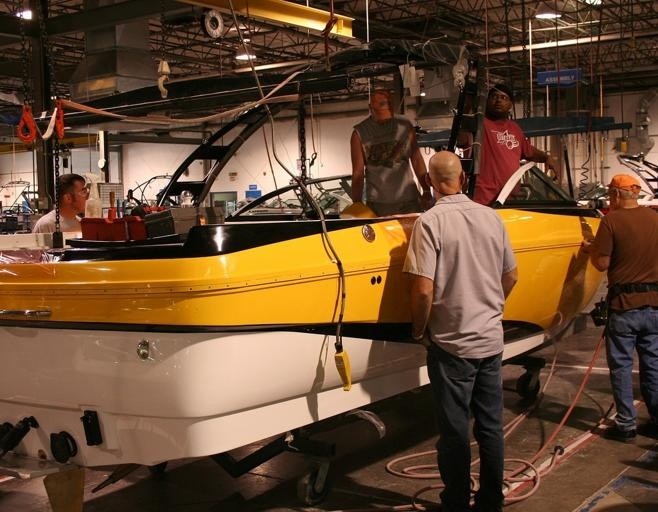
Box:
[603,424,636,442]
[636,420,658,439]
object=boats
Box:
[0,161,609,481]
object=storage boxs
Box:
[80,207,225,240]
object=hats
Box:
[602,174,641,194]
[369,89,396,123]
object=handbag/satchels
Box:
[588,298,612,326]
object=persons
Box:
[402,149,519,512]
[33,172,88,232]
[351,93,434,216]
[457,82,559,206]
[582,173,658,440]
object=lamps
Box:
[235,39,257,61]
[157,59,171,76]
[534,1,562,19]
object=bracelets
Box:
[422,189,430,192]
[412,333,423,342]
